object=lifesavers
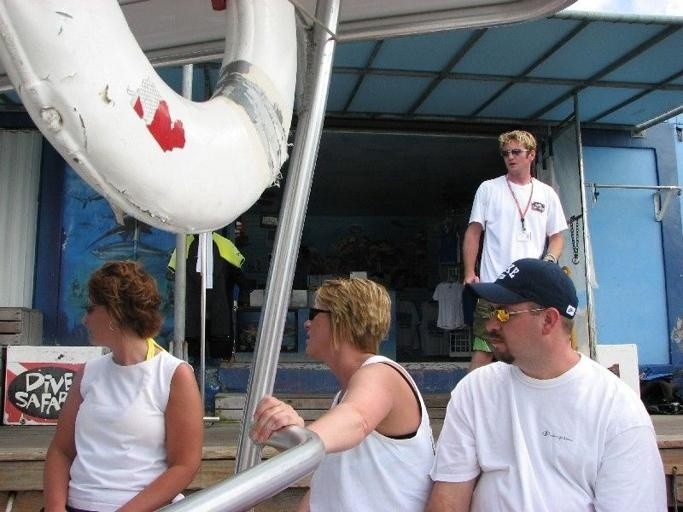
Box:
[0,0,298,235]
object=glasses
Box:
[81,304,96,314]
[308,306,331,320]
[500,149,530,157]
[494,306,548,322]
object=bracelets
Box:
[542,253,556,263]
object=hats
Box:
[466,257,578,319]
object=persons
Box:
[420,259,666,512]
[246,277,435,512]
[42,261,203,512]
[460,130,571,373]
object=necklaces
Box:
[503,174,535,231]
